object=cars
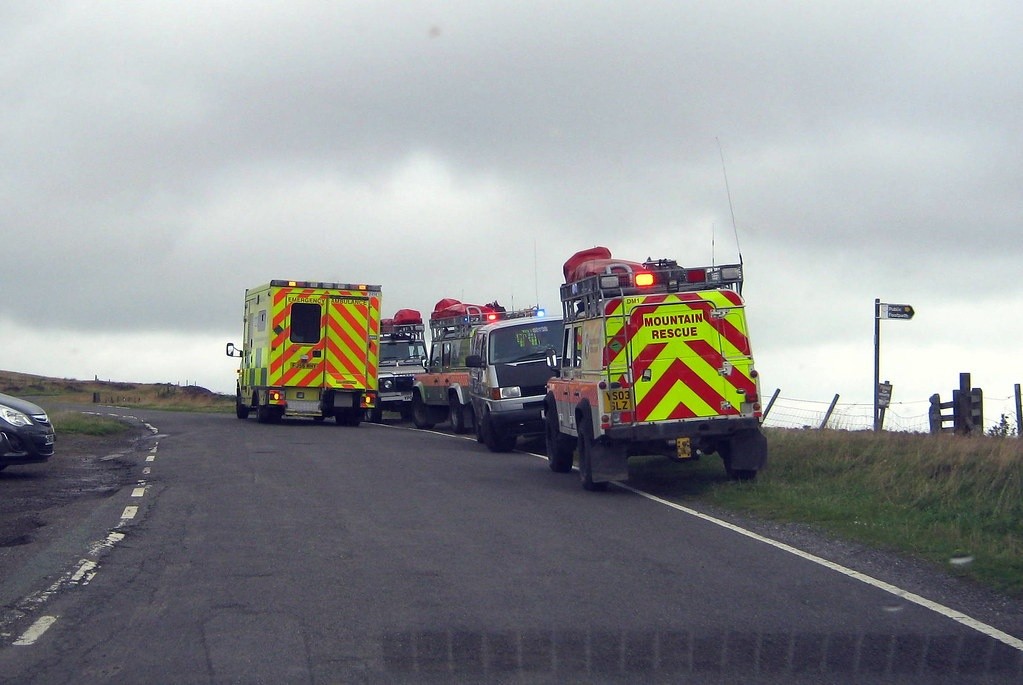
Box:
[0,392,57,471]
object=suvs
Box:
[358,309,429,423]
[409,299,510,434]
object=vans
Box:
[464,307,568,453]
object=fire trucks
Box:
[540,247,769,489]
[226,279,382,426]
